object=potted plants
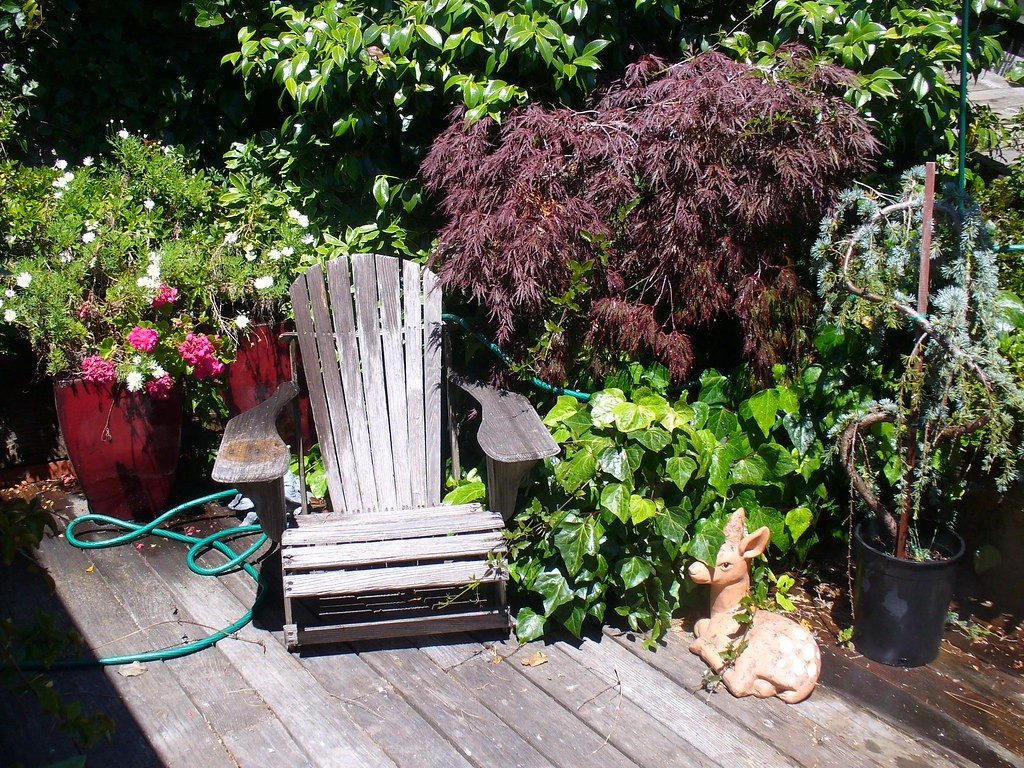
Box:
[808,165,1024,670]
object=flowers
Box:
[0,117,315,398]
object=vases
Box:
[55,369,204,521]
[217,321,313,453]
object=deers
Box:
[687,506,822,705]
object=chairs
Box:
[211,246,561,653]
